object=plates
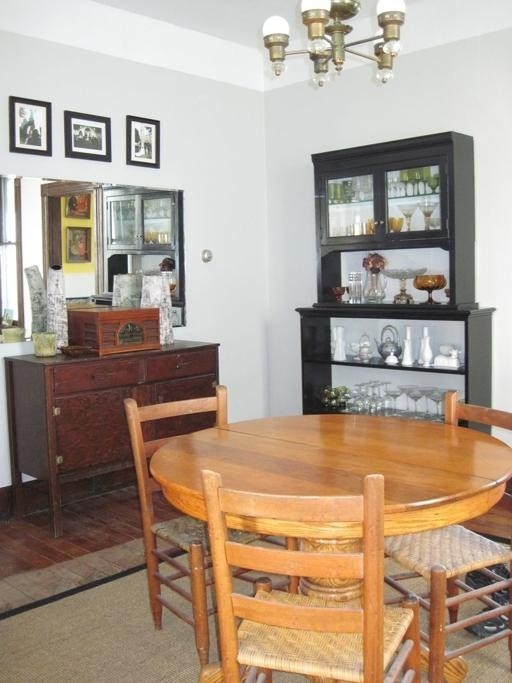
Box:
[56,344,92,357]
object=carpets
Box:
[1,527,511,682]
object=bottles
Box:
[348,271,363,304]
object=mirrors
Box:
[0,176,186,342]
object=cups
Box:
[392,216,405,232]
[157,232,170,244]
[30,331,57,357]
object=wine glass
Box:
[331,286,346,302]
[397,200,442,233]
[327,173,375,204]
[386,165,441,197]
[379,266,447,304]
[345,379,447,422]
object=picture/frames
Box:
[8,96,162,169]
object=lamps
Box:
[260,0,407,87]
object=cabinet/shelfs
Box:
[295,306,497,436]
[309,131,476,308]
[98,188,183,310]
[2,340,220,539]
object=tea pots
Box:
[358,333,377,362]
[373,323,403,360]
